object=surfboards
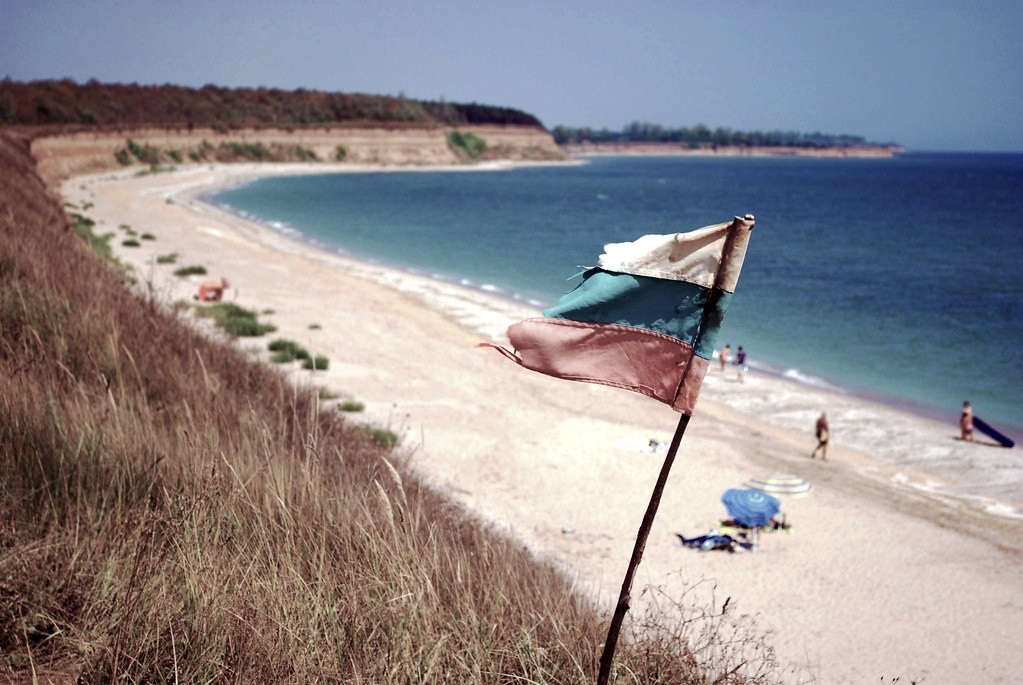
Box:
[971,416,1015,448]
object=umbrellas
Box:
[721,488,780,551]
[741,472,815,499]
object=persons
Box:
[811,411,829,462]
[960,400,973,441]
[732,346,746,383]
[720,345,731,371]
